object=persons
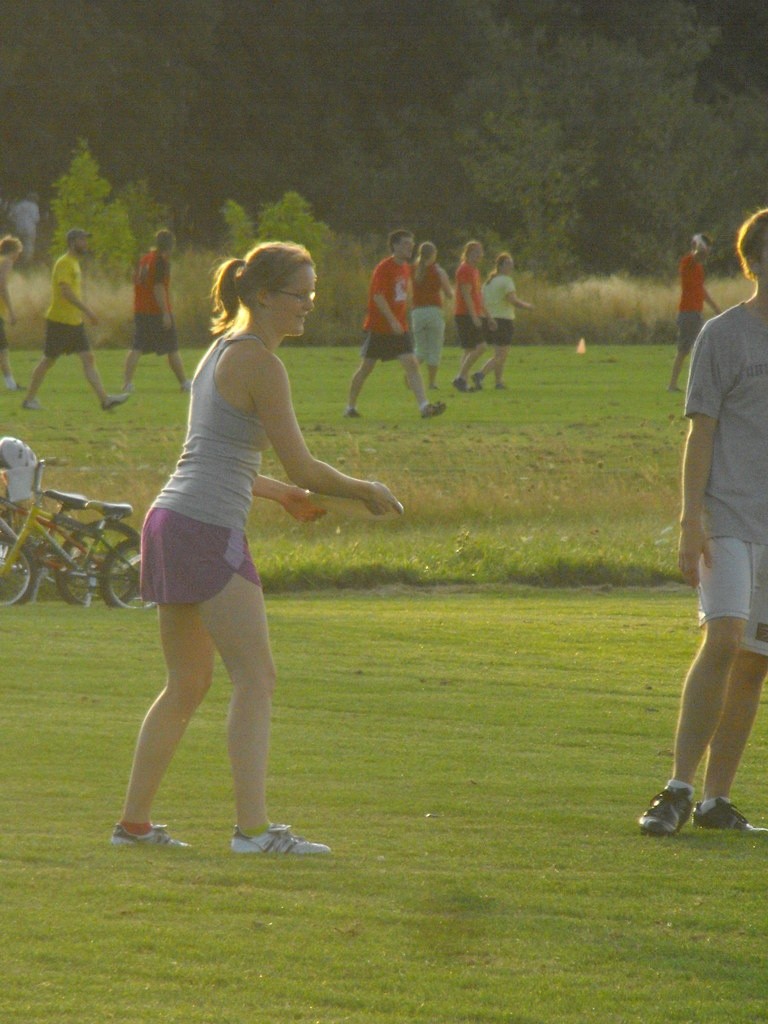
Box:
[666,234,721,392]
[112,242,404,856]
[0,231,130,411]
[344,229,535,418]
[120,230,193,397]
[638,209,768,837]
[9,193,40,269]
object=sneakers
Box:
[692,797,768,834]
[111,821,190,849]
[22,399,44,412]
[639,786,693,835]
[102,394,130,408]
[8,383,26,392]
[231,823,331,854]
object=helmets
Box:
[1,436,38,471]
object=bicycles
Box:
[1,458,159,614]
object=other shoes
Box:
[494,381,508,391]
[420,402,445,418]
[344,406,361,418]
[122,384,135,392]
[472,373,483,390]
[451,376,470,392]
[180,380,192,393]
[667,386,683,393]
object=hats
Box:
[66,229,92,243]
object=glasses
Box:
[263,288,316,303]
